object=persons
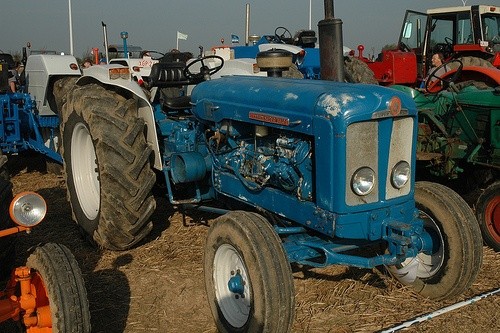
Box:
[426,53,452,87]
[70,62,78,69]
[80,57,107,68]
[7,60,22,93]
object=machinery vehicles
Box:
[0,1,500,333]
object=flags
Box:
[178,32,188,39]
